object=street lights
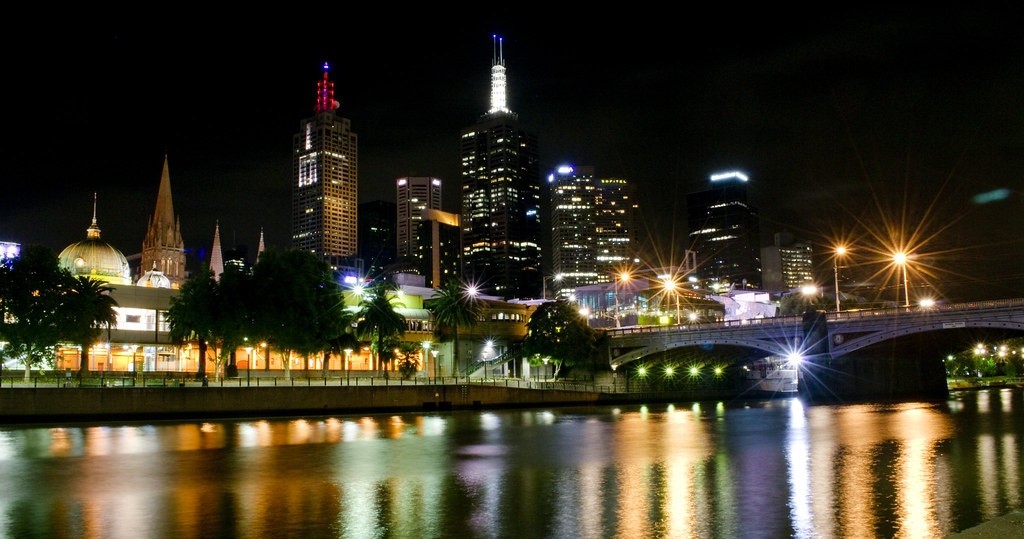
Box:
[830,241,851,318]
[895,251,912,313]
